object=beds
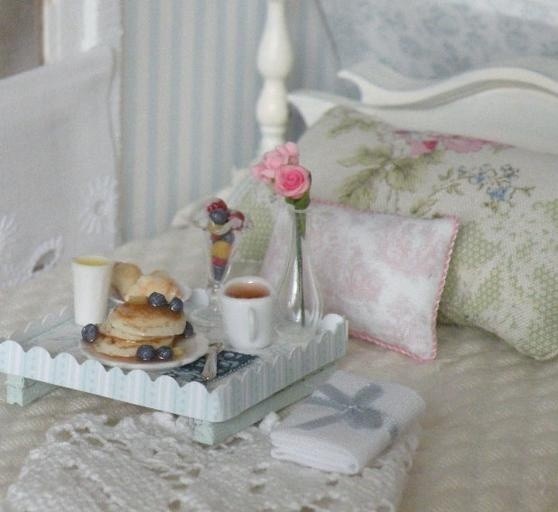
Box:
[0,1,558,511]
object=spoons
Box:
[200,341,226,382]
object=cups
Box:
[71,254,115,326]
[216,275,277,352]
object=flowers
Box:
[247,140,316,329]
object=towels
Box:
[268,367,427,476]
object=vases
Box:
[274,202,325,330]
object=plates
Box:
[77,330,210,370]
[110,280,193,308]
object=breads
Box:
[93,262,189,357]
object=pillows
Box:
[258,195,462,364]
[186,103,557,363]
